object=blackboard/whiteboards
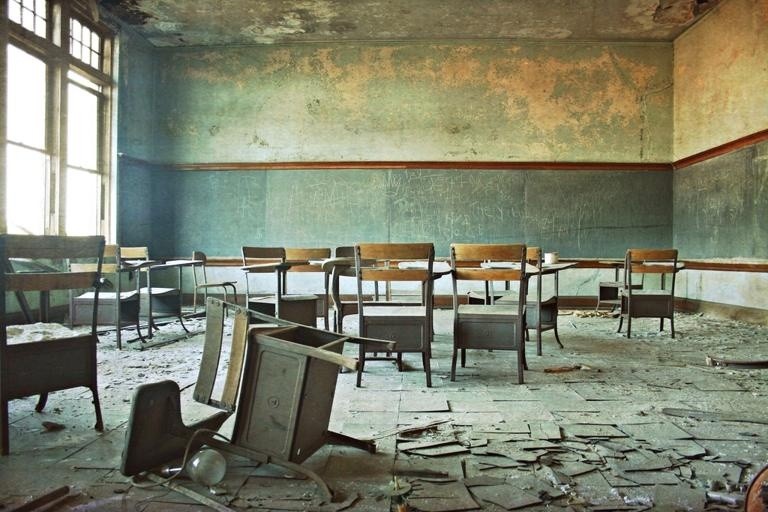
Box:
[117,129,766,272]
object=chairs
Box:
[488,246,566,357]
[595,257,646,312]
[449,242,528,385]
[192,251,238,318]
[616,248,678,338]
[241,245,317,328]
[120,295,399,510]
[283,246,331,330]
[466,267,511,304]
[1,233,105,456]
[353,242,435,388]
[331,246,379,336]
[65,244,146,350]
[120,247,189,335]
